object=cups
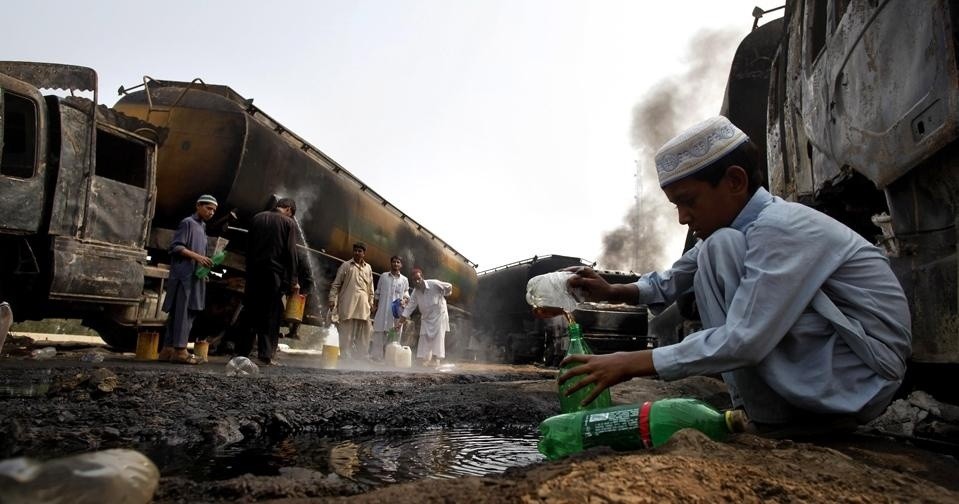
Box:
[520,271,586,318]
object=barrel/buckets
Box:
[395,345,412,369]
[385,341,402,367]
[321,345,339,368]
[285,294,306,322]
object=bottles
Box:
[533,395,751,462]
[195,248,227,278]
[558,320,611,410]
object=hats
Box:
[655,115,749,188]
[197,195,218,207]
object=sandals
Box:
[159,353,199,365]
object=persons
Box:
[369,256,410,361]
[161,195,218,364]
[235,198,315,365]
[396,269,452,368]
[558,115,912,440]
[329,241,375,368]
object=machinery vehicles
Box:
[675,0,958,433]
[477,254,650,368]
[0,51,481,364]
